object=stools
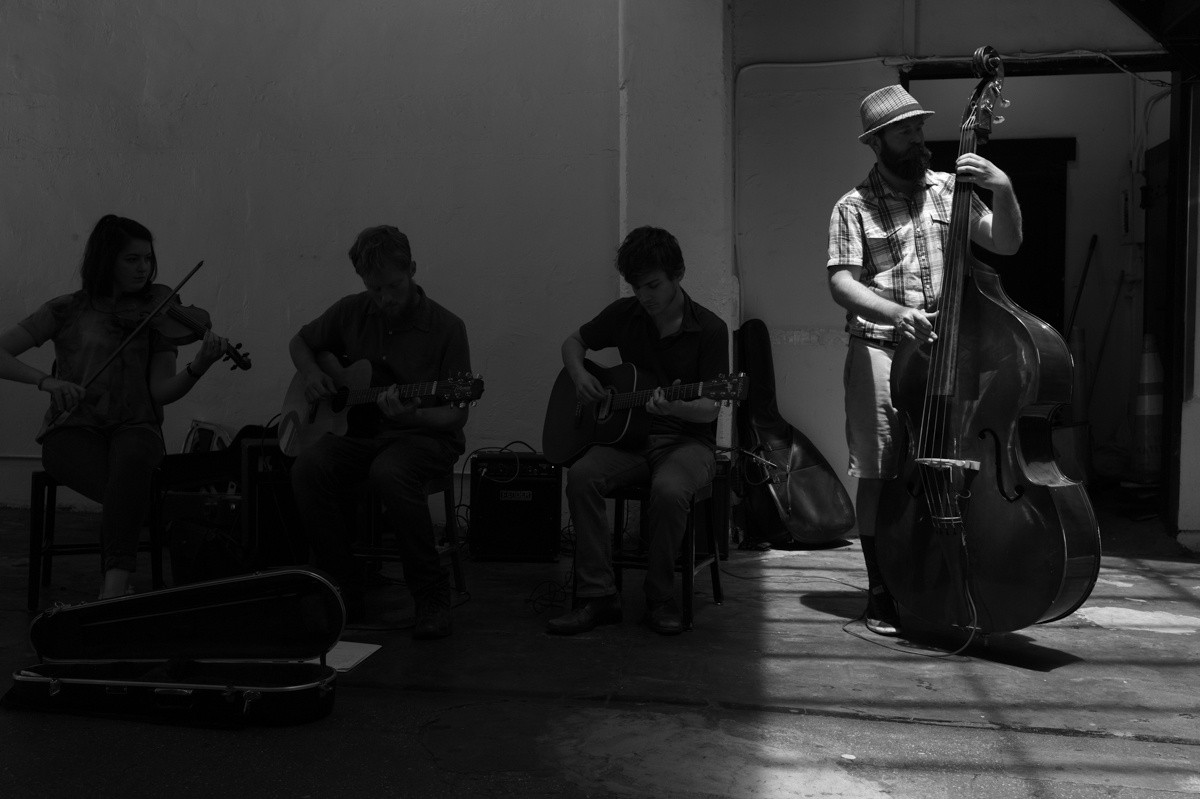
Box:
[349,460,467,595]
[603,480,723,629]
[25,471,163,621]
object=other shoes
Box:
[548,596,621,636]
[867,582,893,618]
[638,606,683,636]
[415,594,455,639]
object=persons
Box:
[826,84,1022,633]
[289,225,471,642]
[546,222,728,635]
[0,214,227,608]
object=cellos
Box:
[874,44,1103,650]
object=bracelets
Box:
[186,362,203,378]
[38,375,52,390]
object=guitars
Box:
[276,349,485,458]
[541,357,751,468]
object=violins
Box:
[110,283,252,372]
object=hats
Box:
[860,83,932,144]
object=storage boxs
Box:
[639,460,731,560]
